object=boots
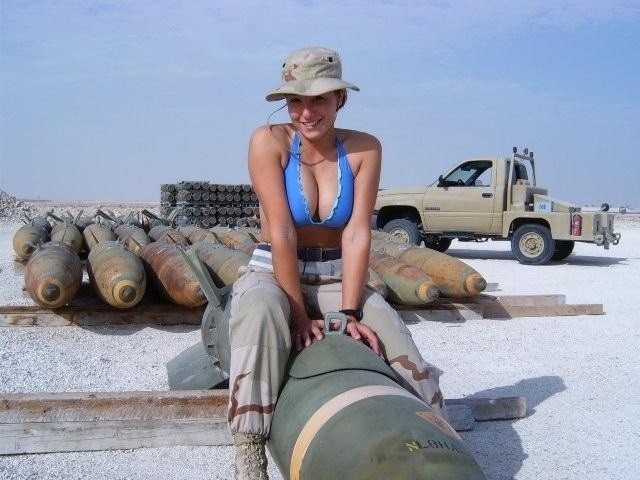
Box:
[234,433,270,480]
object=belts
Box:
[258,243,341,262]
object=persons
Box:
[226,46,445,480]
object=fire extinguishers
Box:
[572,214,581,236]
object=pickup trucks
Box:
[374,147,620,264]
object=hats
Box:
[266,47,360,101]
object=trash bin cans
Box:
[619,207,627,213]
